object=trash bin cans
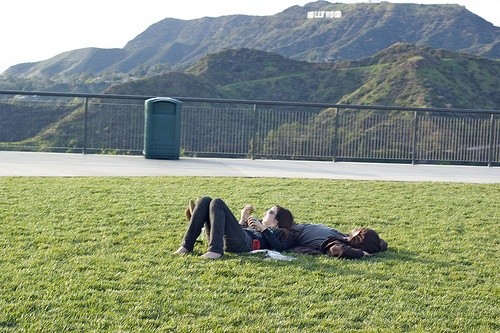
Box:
[142,96,183,161]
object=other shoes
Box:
[198,251,225,259]
[174,246,192,254]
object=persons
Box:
[184,199,388,260]
[173,196,296,259]
